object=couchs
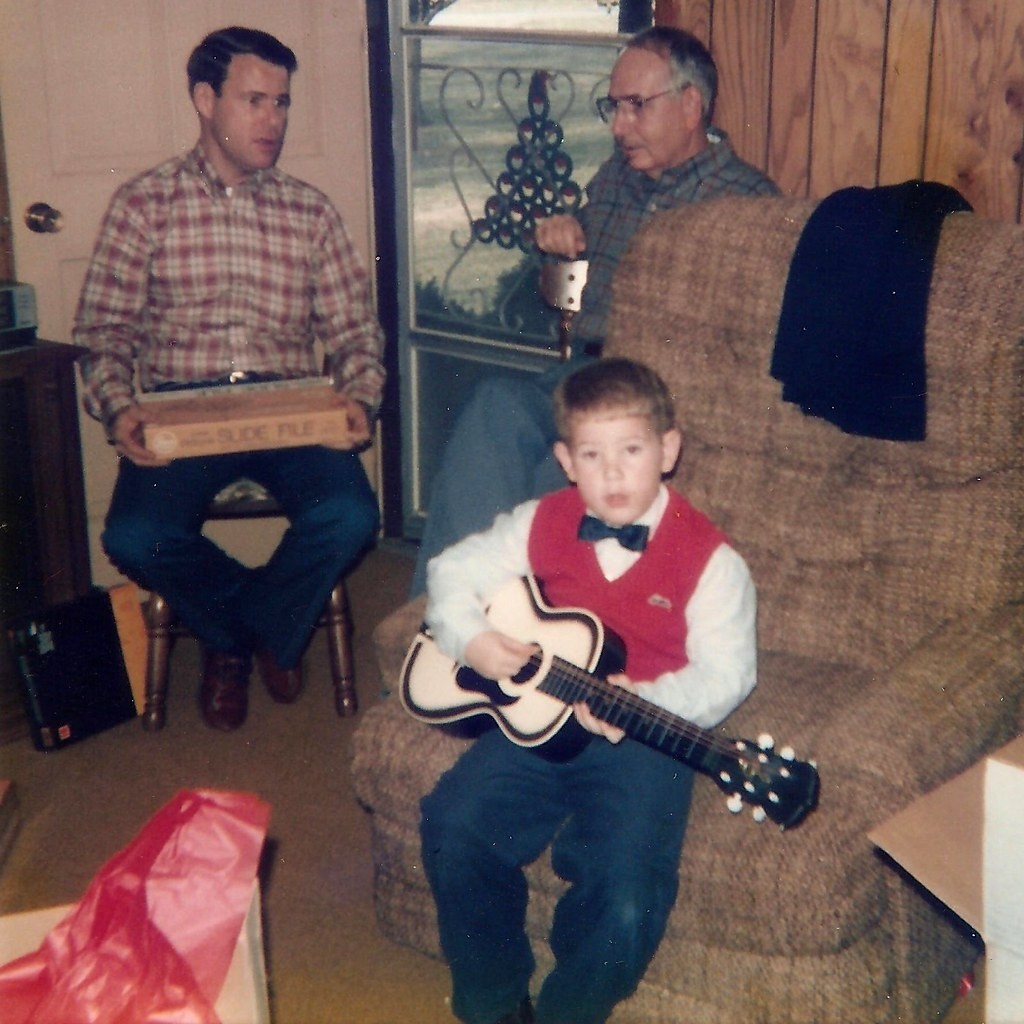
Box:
[359,187,1023,1023]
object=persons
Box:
[69,25,388,733]
[406,26,783,600]
[417,354,759,1024]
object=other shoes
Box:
[198,643,255,732]
[256,648,304,704]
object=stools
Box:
[142,475,360,722]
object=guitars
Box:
[398,572,822,836]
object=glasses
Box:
[595,85,690,125]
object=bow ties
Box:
[576,514,650,553]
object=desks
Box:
[0,334,101,749]
